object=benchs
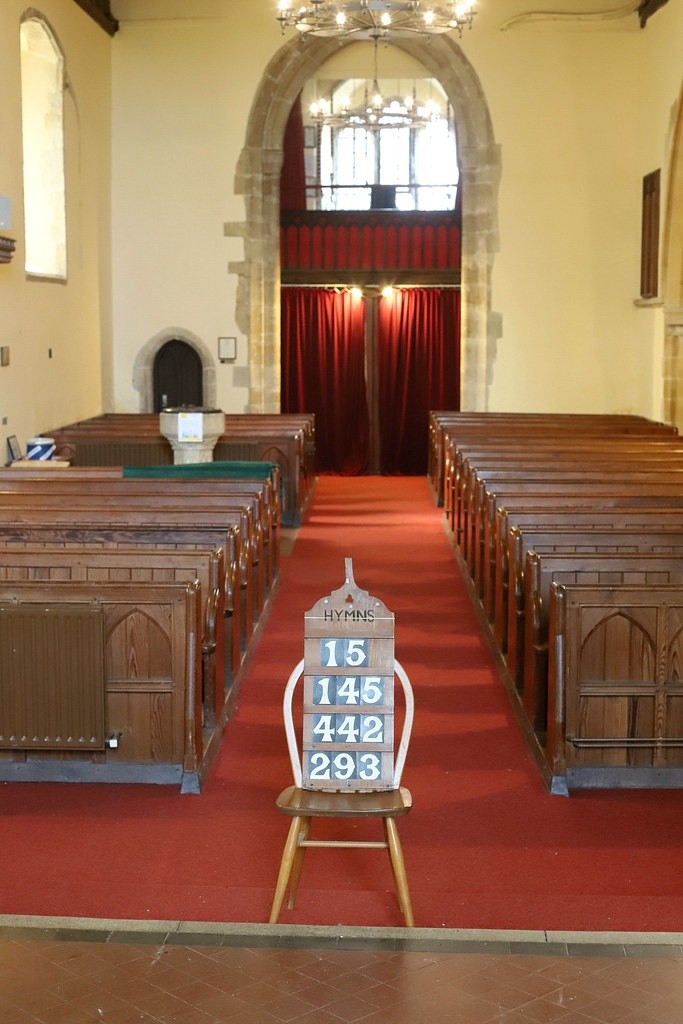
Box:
[428,412,683,797]
[0,411,316,795]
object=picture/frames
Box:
[6,434,22,461]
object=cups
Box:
[25,436,56,461]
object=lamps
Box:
[276,0,480,49]
[308,49,441,130]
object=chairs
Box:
[268,655,416,928]
